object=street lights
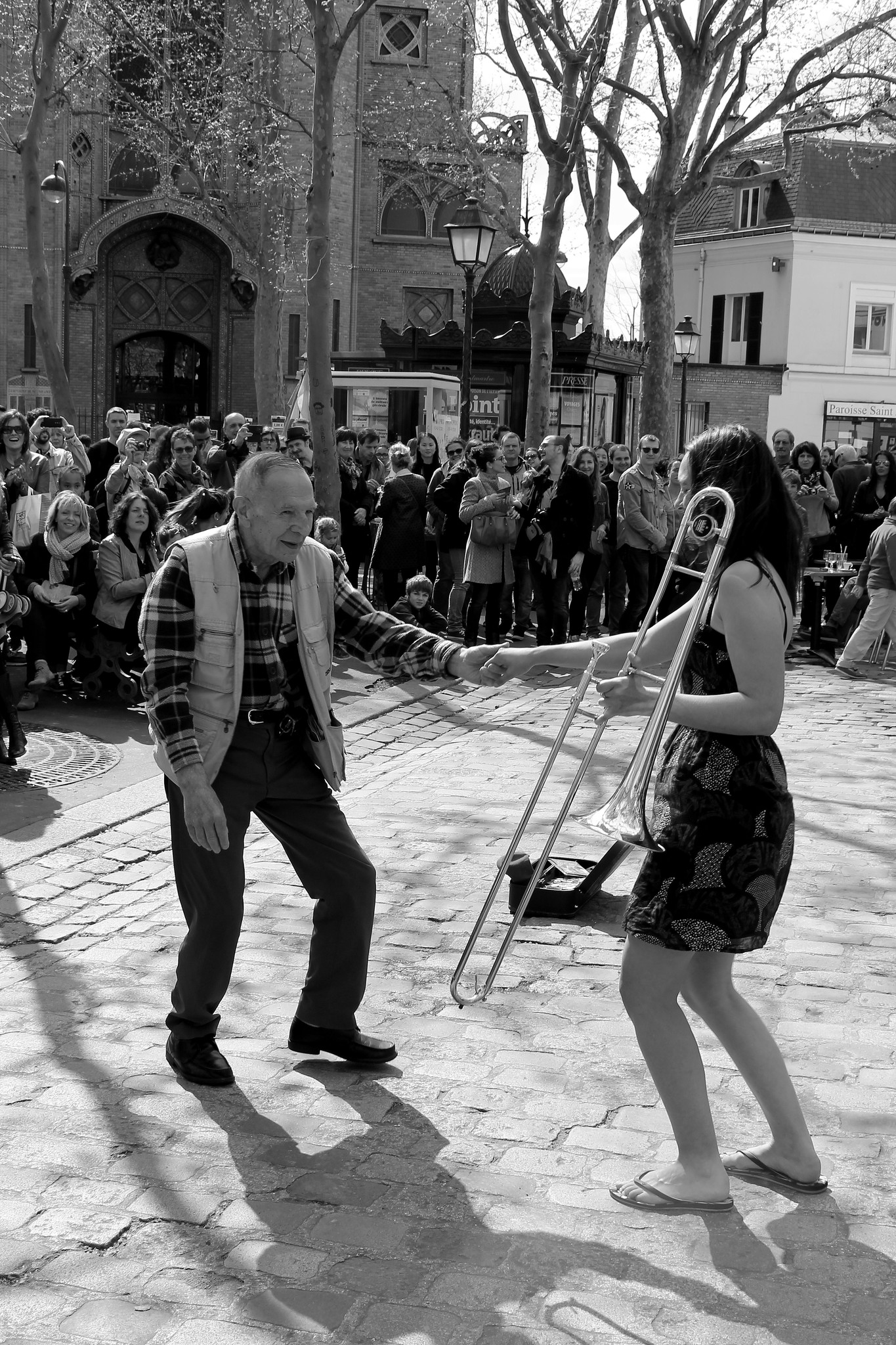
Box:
[674,316,701,453]
[444,196,501,441]
[40,160,71,383]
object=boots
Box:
[1,639,83,766]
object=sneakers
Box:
[447,619,608,647]
[787,626,840,654]
[333,643,350,660]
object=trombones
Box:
[447,482,736,1006]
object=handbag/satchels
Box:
[587,531,604,556]
[39,580,78,609]
[470,477,555,551]
[671,540,699,594]
[424,512,439,542]
[818,468,844,535]
[363,522,377,549]
[8,485,51,551]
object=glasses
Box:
[640,447,660,454]
[148,438,156,443]
[174,436,210,454]
[262,438,277,443]
[375,452,388,459]
[446,443,561,463]
[2,425,26,434]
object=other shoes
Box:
[835,666,868,678]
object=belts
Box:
[238,707,304,724]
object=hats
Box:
[116,428,149,455]
[285,426,311,443]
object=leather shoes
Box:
[166,1033,235,1086]
[288,1017,398,1064]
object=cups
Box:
[836,553,848,571]
[843,562,853,570]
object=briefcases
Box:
[510,840,636,918]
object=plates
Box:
[841,568,856,571]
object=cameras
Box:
[805,488,817,495]
[132,441,146,451]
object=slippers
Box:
[720,1150,828,1194]
[609,1169,734,1211]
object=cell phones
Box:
[247,425,263,433]
[44,417,62,428]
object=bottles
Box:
[570,565,583,592]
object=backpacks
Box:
[87,474,131,538]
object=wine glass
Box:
[823,549,831,569]
[827,552,837,572]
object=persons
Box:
[0,404,896,775]
[484,422,829,1212]
[138,452,506,1089]
